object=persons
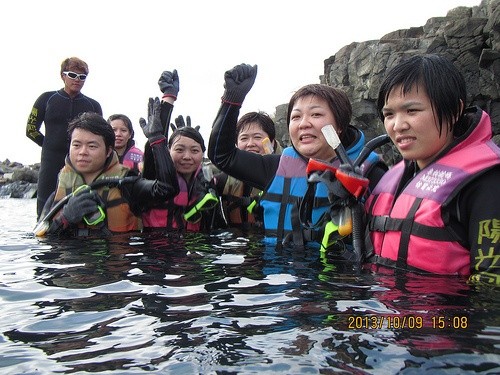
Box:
[30,63,500,285]
[25,58,116,223]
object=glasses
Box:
[73,184,106,228]
[183,186,220,225]
[247,190,265,214]
[63,71,87,82]
[320,221,347,252]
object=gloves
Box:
[221,63,258,106]
[170,115,200,132]
[59,187,99,229]
[158,69,180,101]
[139,97,166,147]
